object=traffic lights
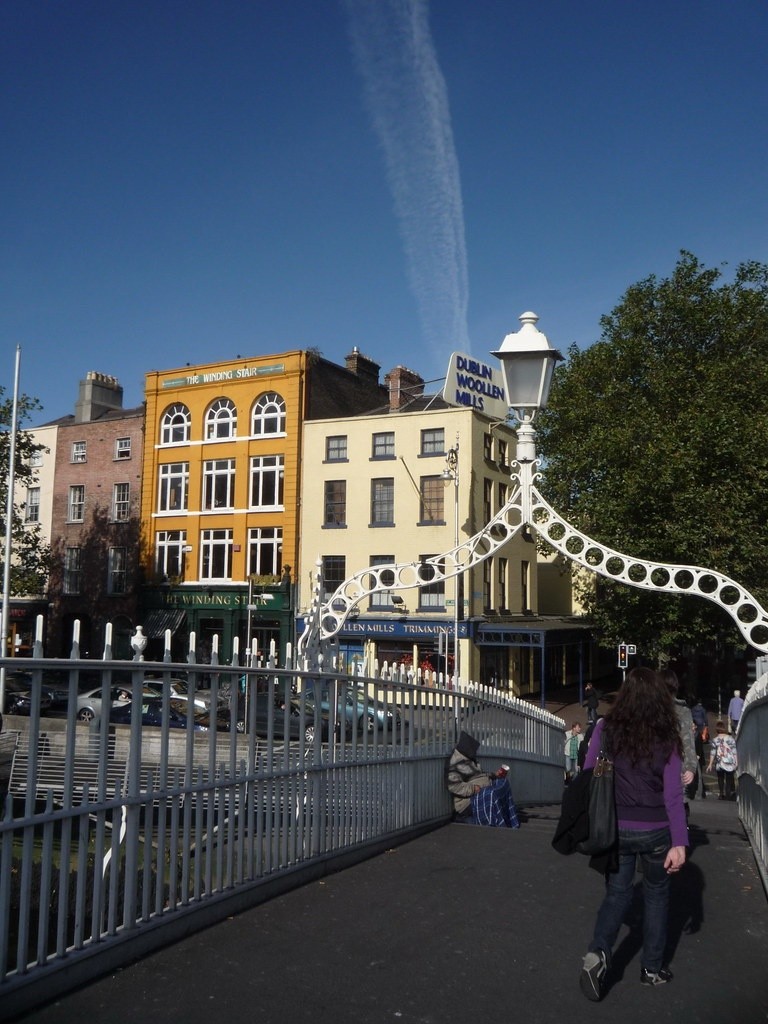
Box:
[618,643,629,670]
[626,644,637,655]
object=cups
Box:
[500,764,509,778]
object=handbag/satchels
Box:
[550,760,619,855]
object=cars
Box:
[297,687,403,734]
[107,694,231,733]
[4,669,69,716]
[74,682,168,722]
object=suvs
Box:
[236,691,351,745]
[143,678,229,714]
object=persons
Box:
[119,691,131,702]
[447,731,500,818]
[280,702,285,709]
[565,667,743,1003]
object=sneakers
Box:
[639,966,673,987]
[579,948,608,1002]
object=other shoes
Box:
[719,795,724,800]
